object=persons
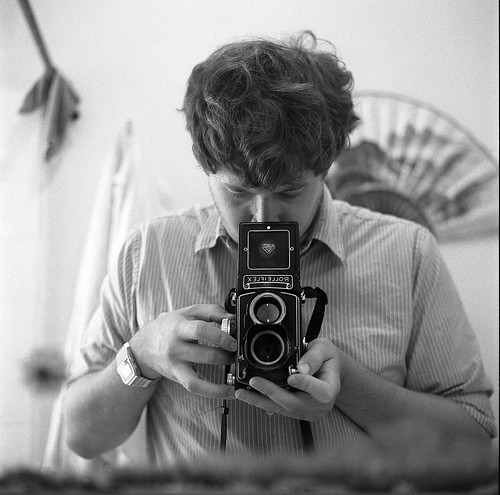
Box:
[61,30,500,492]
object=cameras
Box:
[221,220,308,393]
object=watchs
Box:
[116,342,160,388]
